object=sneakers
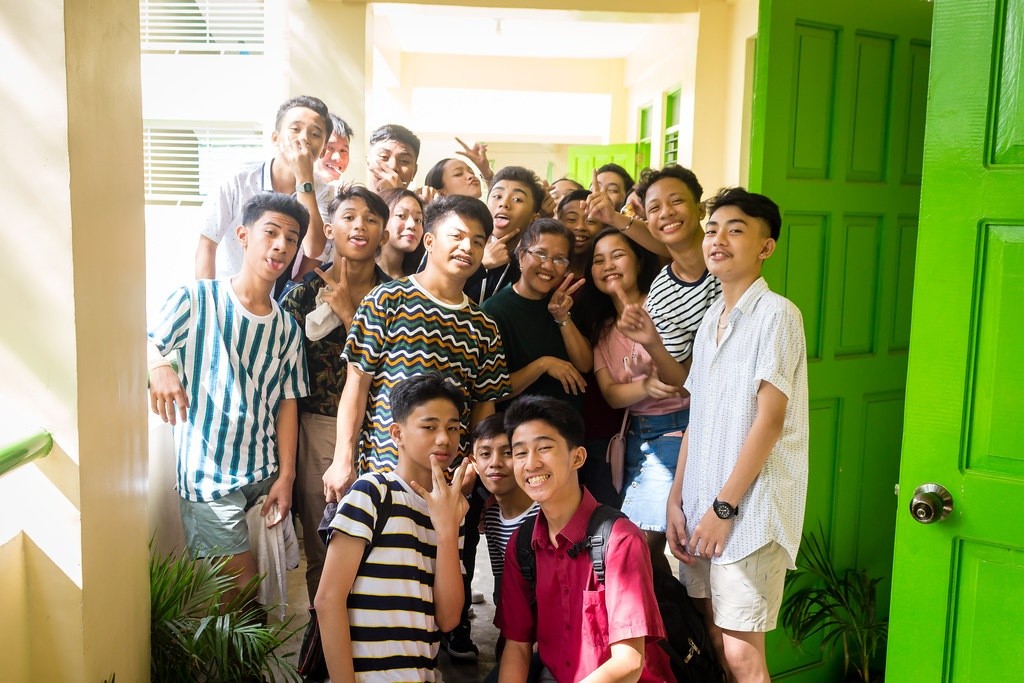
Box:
[445,620,479,659]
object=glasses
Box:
[525,250,570,267]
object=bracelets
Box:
[621,216,634,231]
[480,171,494,182]
[554,312,572,326]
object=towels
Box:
[317,487,350,546]
[244,494,301,623]
[304,285,343,342]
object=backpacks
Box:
[515,503,726,683]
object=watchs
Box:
[296,182,313,192]
[713,497,738,519]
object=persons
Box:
[497,396,677,683]
[666,187,809,683]
[612,165,723,399]
[444,411,543,683]
[313,375,470,683]
[147,95,691,658]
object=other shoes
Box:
[469,605,474,616]
[471,589,485,603]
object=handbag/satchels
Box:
[606,432,627,493]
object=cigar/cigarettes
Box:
[334,168,345,178]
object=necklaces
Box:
[719,323,728,329]
